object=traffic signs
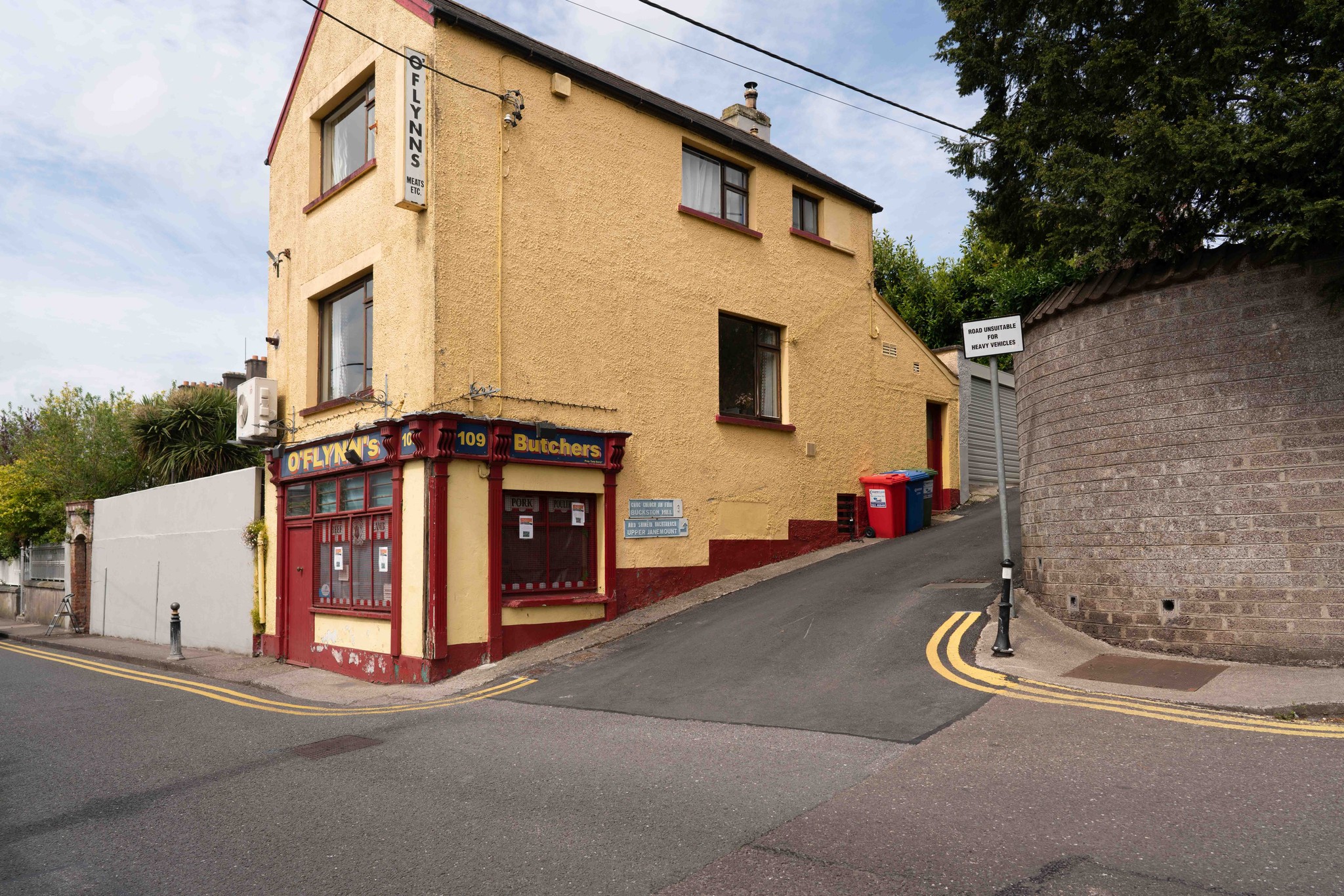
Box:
[960,313,1025,359]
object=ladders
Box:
[45,593,82,636]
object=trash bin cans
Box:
[859,473,911,538]
[905,467,939,528]
[880,469,931,534]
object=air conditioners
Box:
[236,376,278,443]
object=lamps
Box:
[343,422,362,464]
[533,420,558,439]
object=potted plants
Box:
[733,389,755,415]
